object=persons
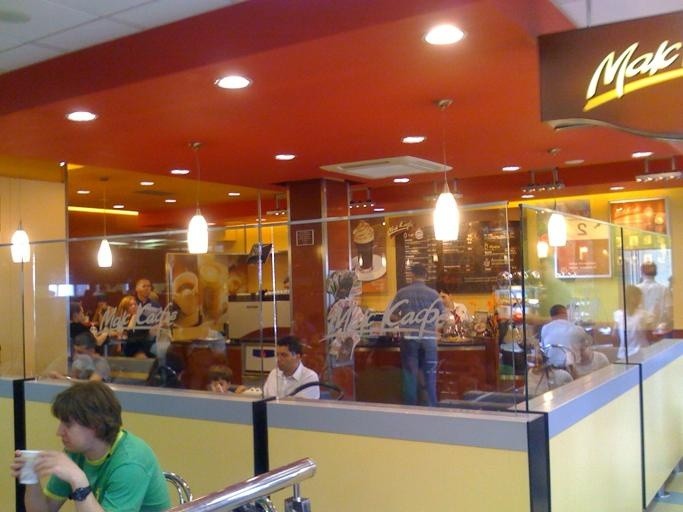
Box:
[437,287,470,326]
[69,278,178,384]
[387,263,447,407]
[7,377,172,511]
[634,260,674,350]
[262,335,320,399]
[540,303,590,380]
[205,363,234,396]
[607,283,658,361]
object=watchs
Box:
[68,485,90,501]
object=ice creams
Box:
[352,220,375,269]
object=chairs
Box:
[159,471,194,510]
[535,342,575,393]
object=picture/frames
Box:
[605,195,674,280]
[552,236,613,281]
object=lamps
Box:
[96,186,113,268]
[431,98,462,242]
[518,166,565,194]
[344,185,375,210]
[634,157,682,185]
[8,177,31,265]
[263,193,288,216]
[543,144,569,249]
[186,142,209,255]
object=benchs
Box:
[67,354,158,385]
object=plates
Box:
[349,253,387,282]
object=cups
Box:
[16,450,44,487]
[353,241,373,274]
